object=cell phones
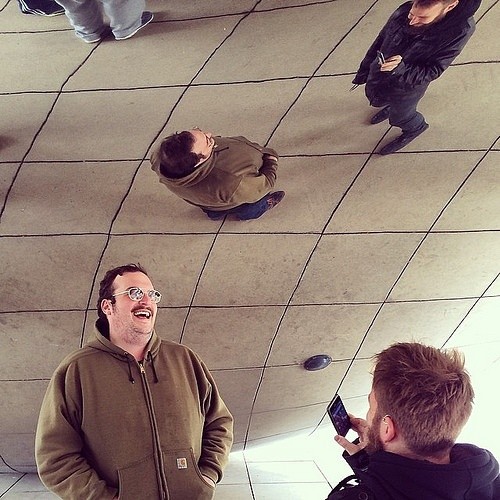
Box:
[377,50,385,66]
[327,394,352,438]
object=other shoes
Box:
[74,30,101,44]
[380,124,429,156]
[114,10,153,40]
[266,191,285,212]
[371,105,391,125]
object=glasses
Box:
[191,126,211,153]
[113,287,163,304]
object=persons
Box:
[150,125,285,221]
[54,0,153,43]
[17,0,65,17]
[351,1,476,155]
[326,343,500,500]
[34,262,236,500]
